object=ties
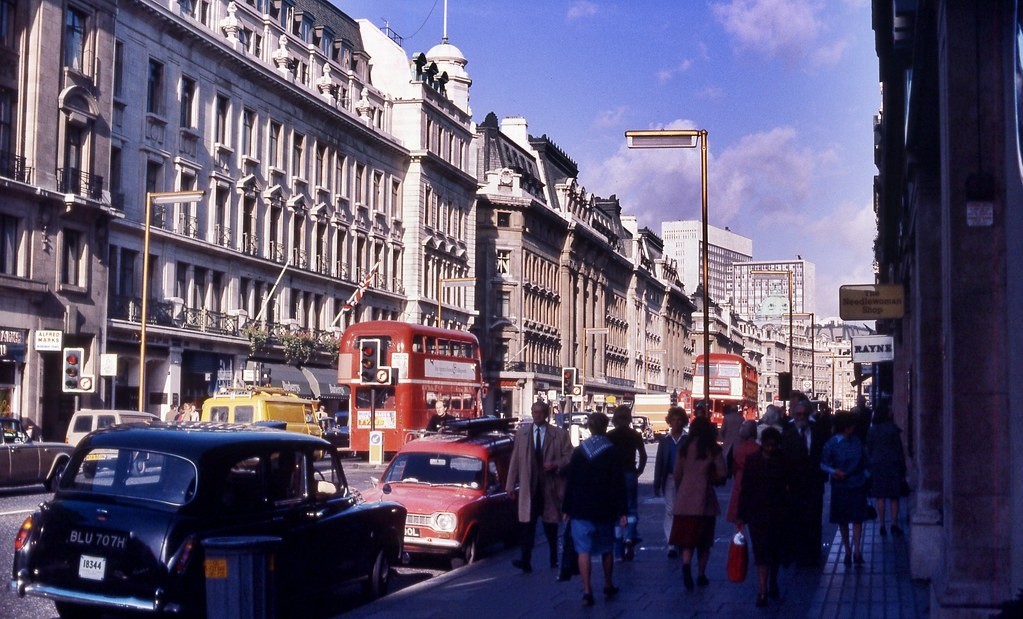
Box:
[535,429,542,465]
[800,425,808,458]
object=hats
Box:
[739,419,755,438]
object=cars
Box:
[359,427,524,569]
[9,421,408,619]
[515,411,614,437]
[1,419,76,490]
[631,416,654,443]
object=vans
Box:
[202,389,330,455]
[66,410,179,476]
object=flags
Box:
[342,264,379,310]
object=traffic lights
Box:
[62,347,97,394]
[562,368,582,396]
[359,339,391,386]
[259,363,272,387]
[672,393,676,405]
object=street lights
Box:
[815,352,834,414]
[750,270,792,385]
[139,191,207,412]
[784,314,815,399]
[645,347,666,395]
[438,277,478,328]
[625,129,709,425]
[582,328,609,411]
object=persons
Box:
[424,399,455,435]
[494,391,911,606]
[318,405,330,433]
[166,402,199,422]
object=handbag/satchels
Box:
[900,478,911,496]
[867,504,878,519]
[560,518,580,580]
[728,523,749,581]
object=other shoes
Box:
[890,524,904,537]
[622,538,642,562]
[604,585,621,601]
[697,575,711,585]
[879,526,887,537]
[557,574,572,582]
[769,585,780,597]
[845,555,851,568]
[580,592,595,606]
[512,551,533,574]
[667,550,680,558]
[682,564,694,593]
[756,591,768,605]
[853,552,864,563]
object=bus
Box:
[678,390,692,419]
[692,353,758,426]
[337,320,486,455]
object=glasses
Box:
[794,412,809,417]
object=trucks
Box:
[633,393,671,435]
